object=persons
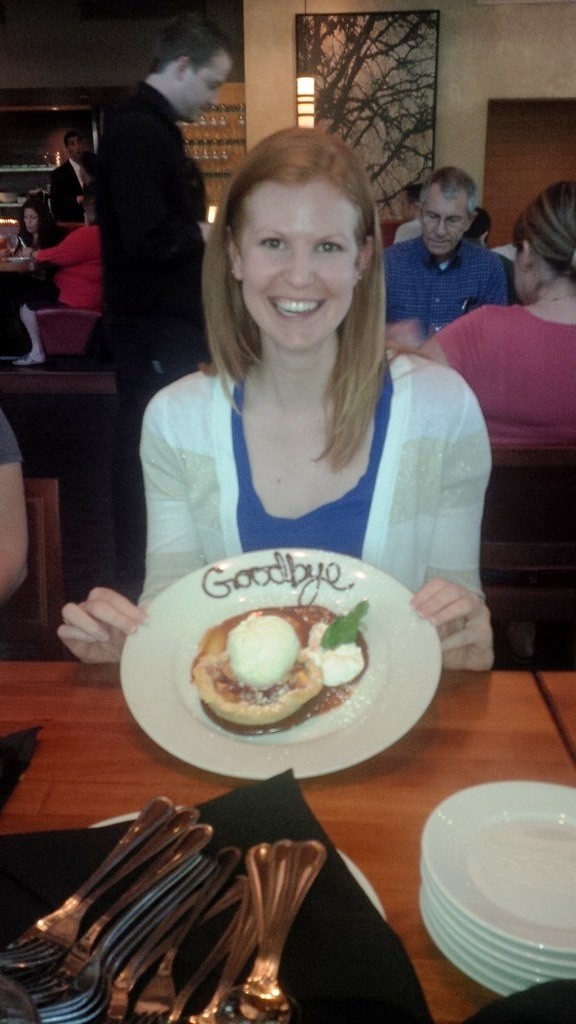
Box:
[58,126,495,671]
[0,135,99,365]
[1,410,28,608]
[377,166,576,450]
[89,14,235,598]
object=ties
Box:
[81,167,88,187]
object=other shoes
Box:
[11,353,48,367]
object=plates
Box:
[118,547,444,779]
[84,812,392,1023]
[6,256,31,263]
[416,780,576,997]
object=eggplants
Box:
[192,651,326,735]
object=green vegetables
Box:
[321,598,369,650]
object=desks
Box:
[0,654,576,1024]
[0,262,35,357]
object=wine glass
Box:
[176,100,247,173]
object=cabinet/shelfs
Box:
[0,102,92,219]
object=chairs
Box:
[478,444,576,670]
[0,309,128,660]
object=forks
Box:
[0,792,247,1024]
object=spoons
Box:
[212,836,326,1023]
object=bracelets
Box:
[29,249,38,257]
